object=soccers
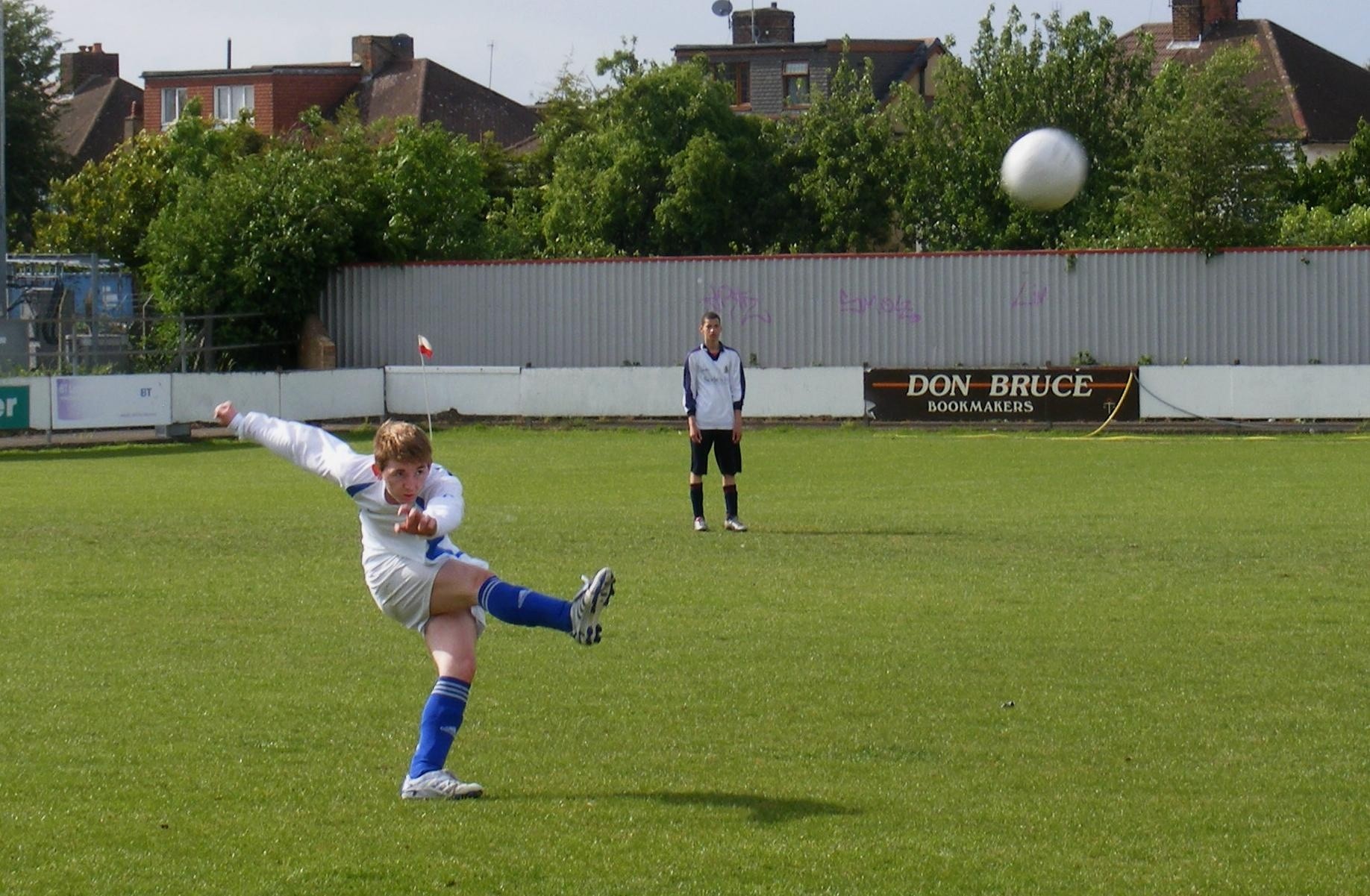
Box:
[999,129,1088,210]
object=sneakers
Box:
[569,567,615,646]
[693,517,707,531]
[400,766,482,801]
[724,517,747,532]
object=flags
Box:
[418,334,433,359]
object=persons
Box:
[681,312,749,531]
[213,401,616,800]
